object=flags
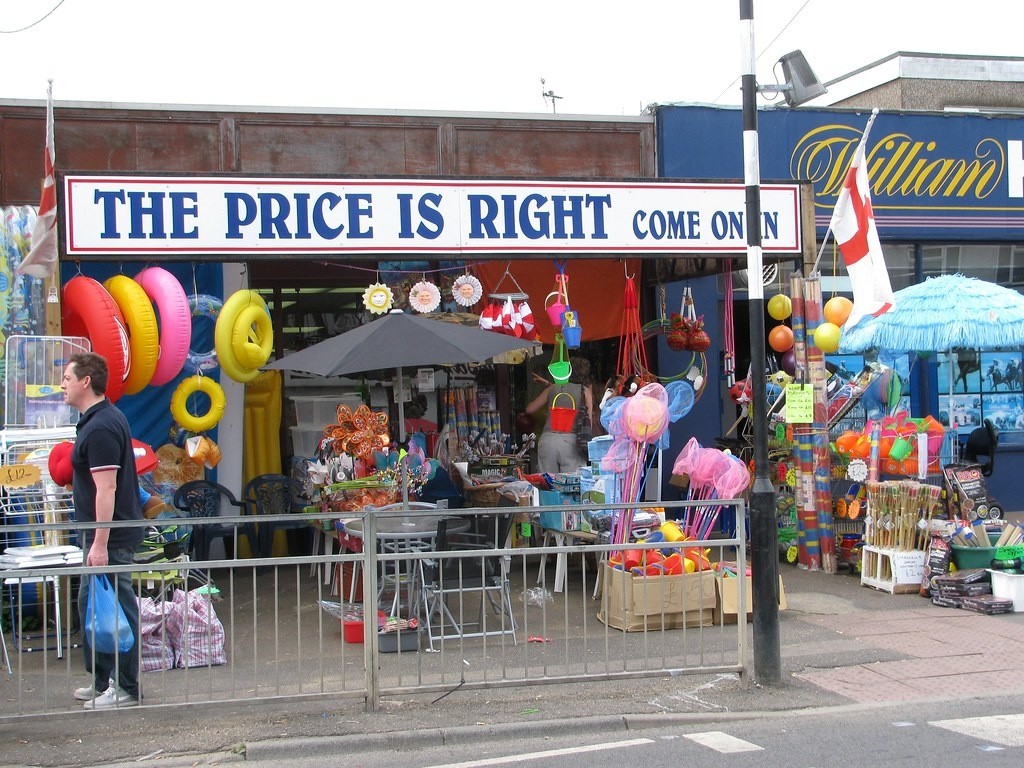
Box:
[16,90,60,278]
[828,118,895,335]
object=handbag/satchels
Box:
[576,384,593,458]
[166,584,227,668]
[136,597,176,673]
[84,574,135,654]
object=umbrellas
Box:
[839,272,1023,470]
[264,309,541,482]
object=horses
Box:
[945,346,1023,391]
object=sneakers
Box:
[74,685,102,701]
[83,677,139,709]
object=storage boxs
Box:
[468,456,530,479]
[985,568,1024,612]
[537,439,644,535]
[596,560,717,633]
[713,576,788,624]
[337,611,388,642]
[931,568,1014,615]
[376,628,426,653]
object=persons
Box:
[533,358,592,452]
[936,346,1022,429]
[59,353,144,711]
[526,357,593,473]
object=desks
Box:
[342,515,471,634]
[513,517,602,600]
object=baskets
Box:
[462,482,507,508]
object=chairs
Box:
[174,479,264,576]
[243,474,315,573]
[410,494,519,652]
[131,525,189,600]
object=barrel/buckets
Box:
[608,520,711,576]
[548,361,572,384]
[545,291,568,326]
[548,392,579,433]
[835,415,953,475]
[562,318,582,349]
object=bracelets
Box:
[545,381,550,387]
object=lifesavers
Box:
[60,265,275,434]
